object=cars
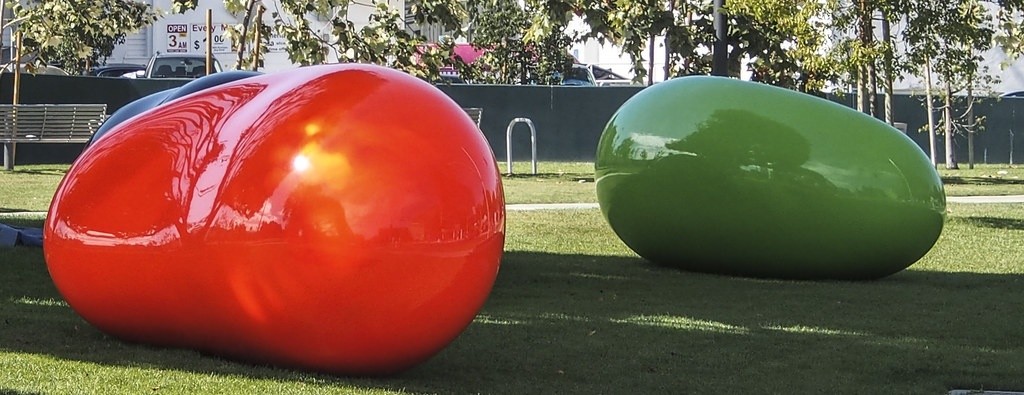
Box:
[523,62,632,86]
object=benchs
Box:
[0,104,107,171]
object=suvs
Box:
[0,51,223,78]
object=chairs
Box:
[157,65,206,75]
